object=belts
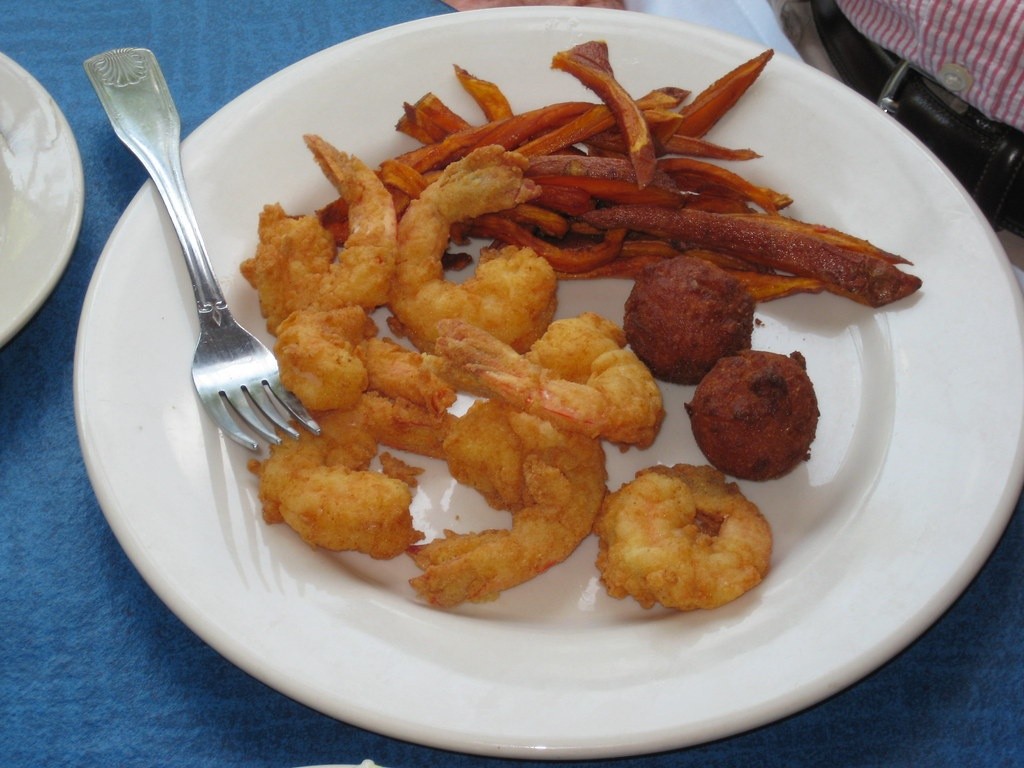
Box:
[810,0,1024,238]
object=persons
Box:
[770,0,1024,230]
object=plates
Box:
[74,4,1024,761]
[0,51,84,355]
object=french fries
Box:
[305,37,925,308]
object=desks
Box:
[1,1,1024,768]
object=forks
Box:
[82,47,323,454]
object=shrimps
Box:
[240,130,774,612]
